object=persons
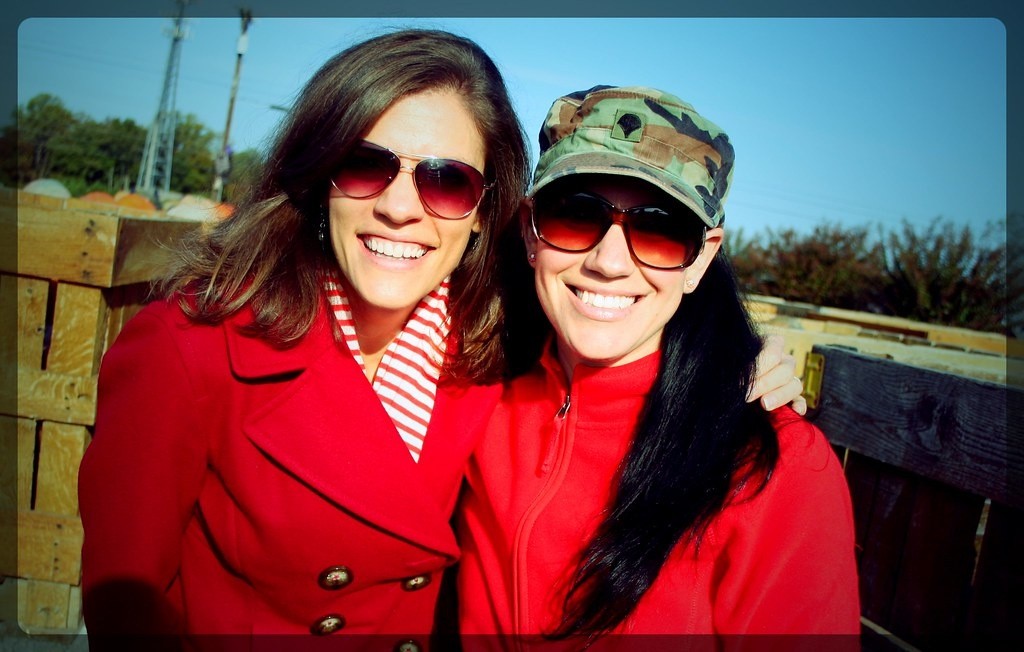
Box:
[449,83,864,652]
[77,28,550,652]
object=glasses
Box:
[530,185,705,271]
[330,138,496,219]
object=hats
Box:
[527,85,736,229]
